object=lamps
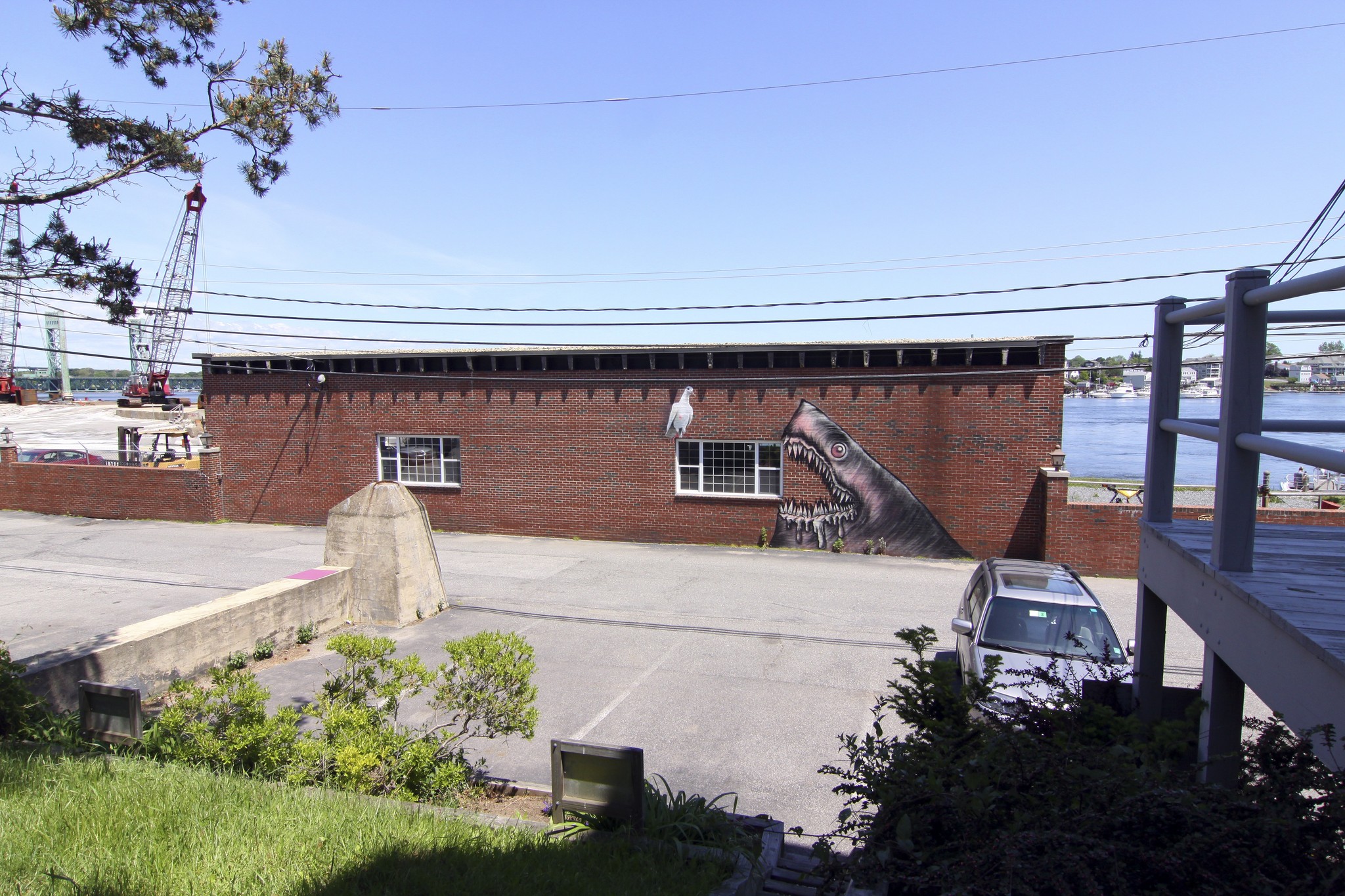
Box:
[0,426,14,443]
[1049,445,1066,471]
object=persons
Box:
[1293,466,1303,489]
[1316,467,1328,480]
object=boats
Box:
[1091,390,1111,398]
[1108,384,1138,398]
[1065,390,1083,396]
[1280,390,1297,393]
[1180,387,1210,398]
[1138,389,1151,397]
[1194,388,1221,398]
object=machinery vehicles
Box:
[0,182,22,404]
[116,183,208,407]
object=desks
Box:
[139,425,192,461]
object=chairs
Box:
[156,449,176,462]
[59,455,66,461]
[72,454,80,459]
[990,609,1028,643]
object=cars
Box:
[951,556,1135,736]
[1109,383,1116,386]
[17,449,108,466]
[381,441,435,466]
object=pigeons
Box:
[664,385,697,439]
